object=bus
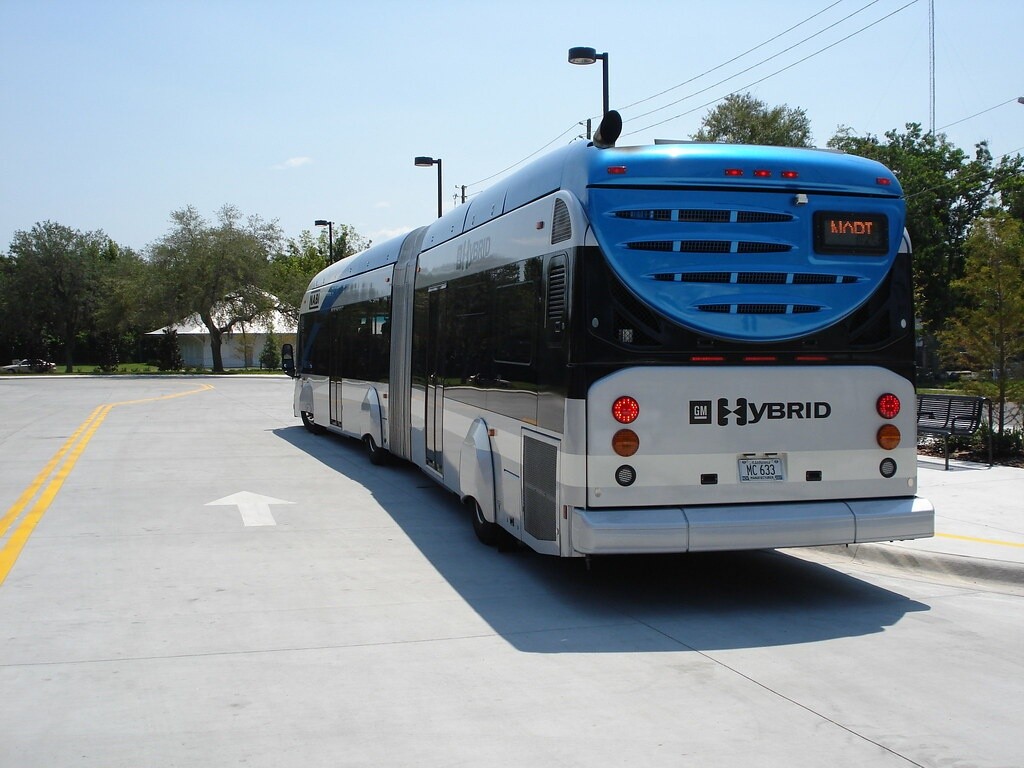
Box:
[278,110,939,583]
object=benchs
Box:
[916,393,994,470]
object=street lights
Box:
[314,220,333,266]
[567,43,611,117]
[416,155,445,222]
